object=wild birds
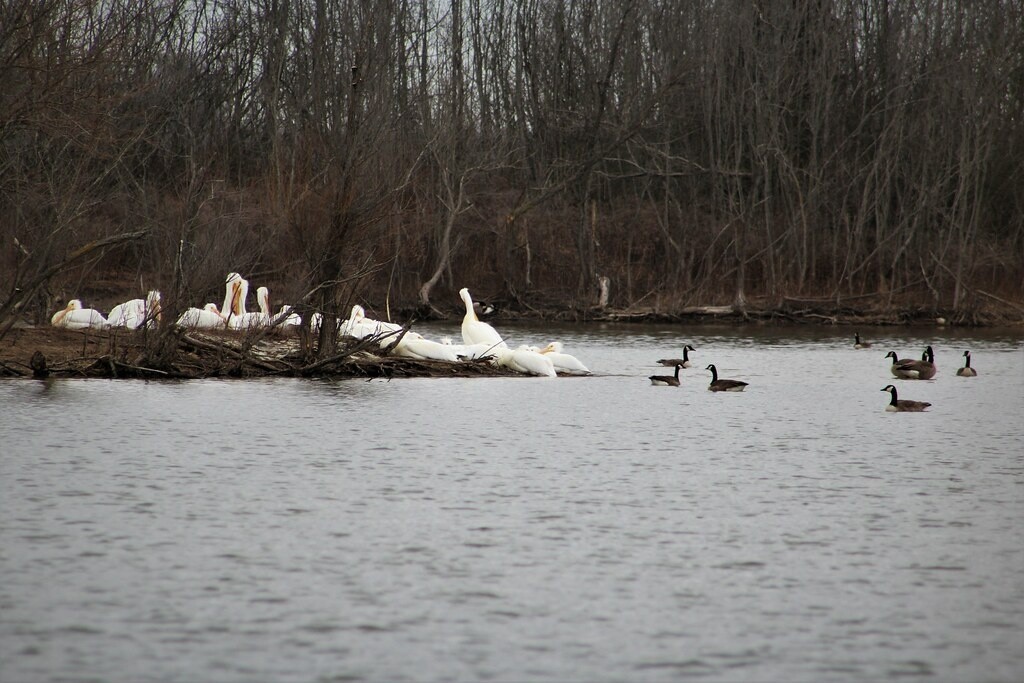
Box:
[884,346,937,380]
[852,330,873,350]
[705,364,750,392]
[880,384,932,413]
[50,272,597,381]
[648,344,696,388]
[955,351,977,377]
